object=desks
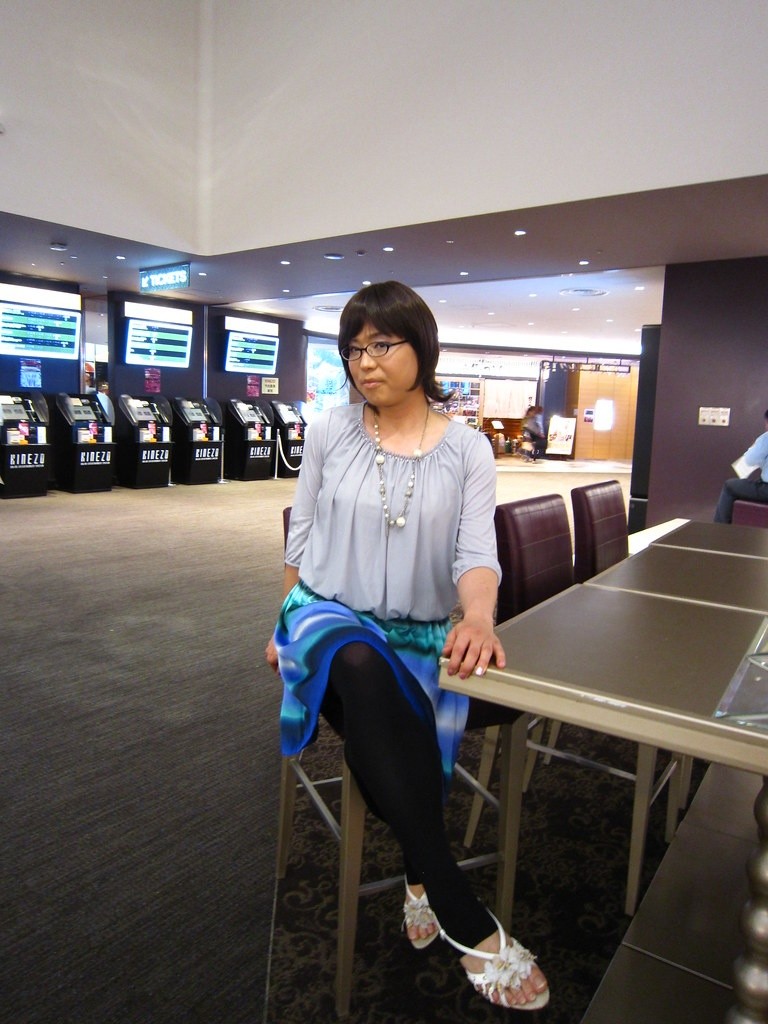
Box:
[432,519,768,1024]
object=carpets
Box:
[265,713,709,1024]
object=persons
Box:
[266,279,549,1010]
[713,409,768,525]
[521,406,546,463]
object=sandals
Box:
[428,897,550,1011]
[405,864,442,949]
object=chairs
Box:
[270,479,691,1024]
[731,500,768,528]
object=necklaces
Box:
[374,403,429,528]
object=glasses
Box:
[340,339,409,362]
